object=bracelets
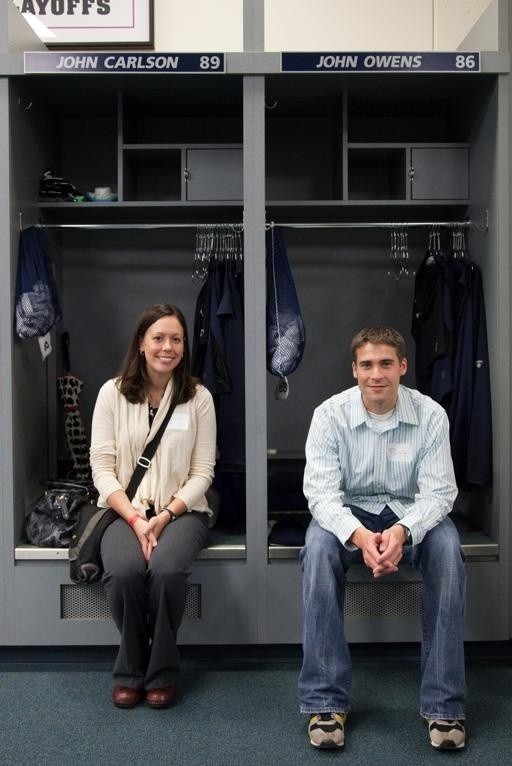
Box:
[128,512,141,529]
[400,523,412,547]
[163,508,177,522]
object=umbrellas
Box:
[54,329,93,499]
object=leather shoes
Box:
[147,684,177,710]
[113,685,143,708]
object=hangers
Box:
[189,222,242,285]
[386,222,470,282]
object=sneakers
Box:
[428,720,466,752]
[307,711,349,751]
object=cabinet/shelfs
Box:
[0,0,512,647]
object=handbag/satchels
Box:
[69,503,120,584]
[23,479,100,549]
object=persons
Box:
[87,301,221,712]
[296,326,470,752]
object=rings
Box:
[393,561,398,566]
